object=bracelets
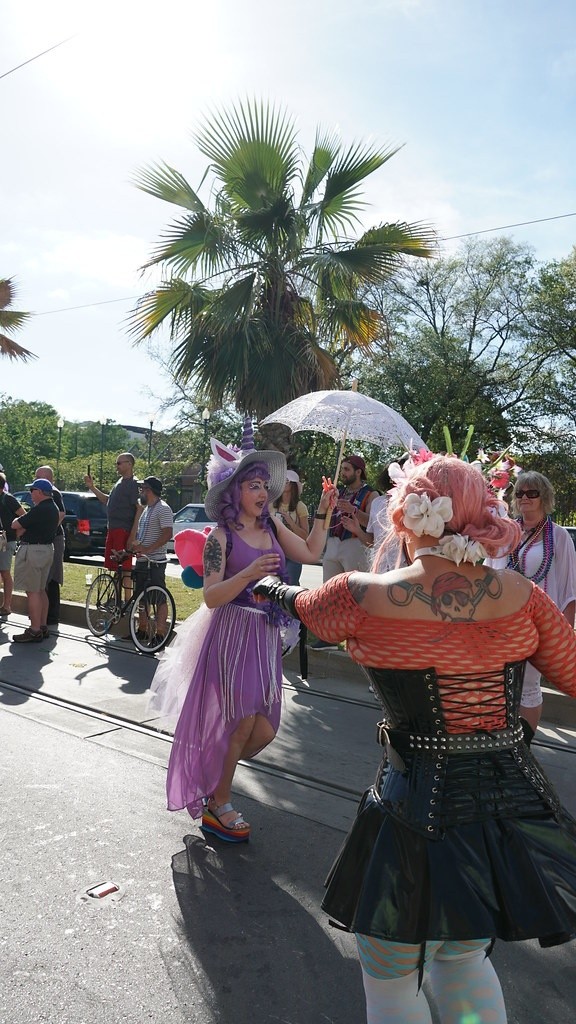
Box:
[313,511,326,520]
[348,504,357,516]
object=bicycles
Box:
[85,550,179,655]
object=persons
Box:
[11,478,60,643]
[85,452,145,612]
[309,456,381,650]
[269,470,310,585]
[36,465,66,625]
[482,470,576,749]
[365,458,408,699]
[0,464,28,618]
[145,409,339,842]
[253,454,576,1024]
[120,476,173,651]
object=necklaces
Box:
[340,481,365,504]
[506,515,554,585]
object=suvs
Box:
[11,491,110,562]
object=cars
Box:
[165,504,219,553]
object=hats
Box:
[136,476,162,491]
[24,479,53,492]
[287,470,302,495]
[203,417,287,522]
[341,456,367,480]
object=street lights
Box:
[147,419,154,464]
[99,418,106,491]
[55,417,65,480]
[201,407,211,482]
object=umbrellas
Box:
[258,377,432,530]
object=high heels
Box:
[201,791,250,842]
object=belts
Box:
[374,719,526,772]
[16,541,53,545]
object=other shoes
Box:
[99,598,125,611]
[134,636,168,653]
[121,631,149,642]
[310,639,339,651]
[112,603,134,615]
[46,624,58,632]
[0,607,12,616]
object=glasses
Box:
[114,460,130,466]
[515,489,539,500]
[139,486,152,491]
[30,488,38,493]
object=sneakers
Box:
[25,626,49,638]
[13,628,43,642]
